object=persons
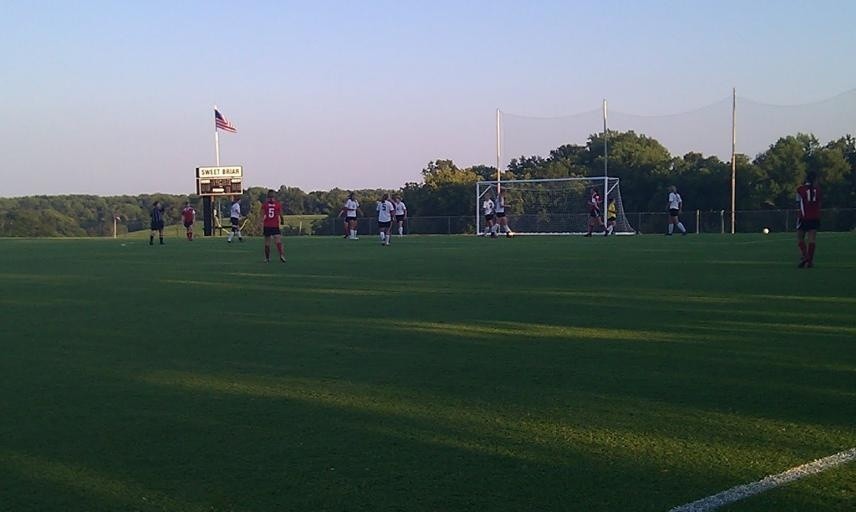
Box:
[482,193,498,238]
[585,188,610,237]
[338,200,348,238]
[182,201,196,241]
[148,199,164,245]
[261,190,287,263]
[793,172,822,266]
[491,191,513,239]
[375,193,407,247]
[226,198,242,243]
[338,191,365,240]
[665,184,688,236]
[607,198,617,234]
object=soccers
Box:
[763,229,769,234]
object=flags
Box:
[216,107,238,134]
